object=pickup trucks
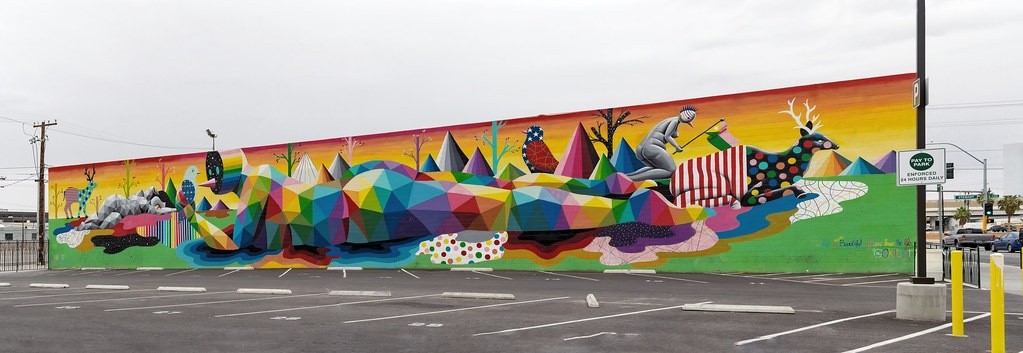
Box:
[943,227,996,251]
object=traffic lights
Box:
[985,202,993,216]
[987,219,994,223]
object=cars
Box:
[994,232,1023,253]
